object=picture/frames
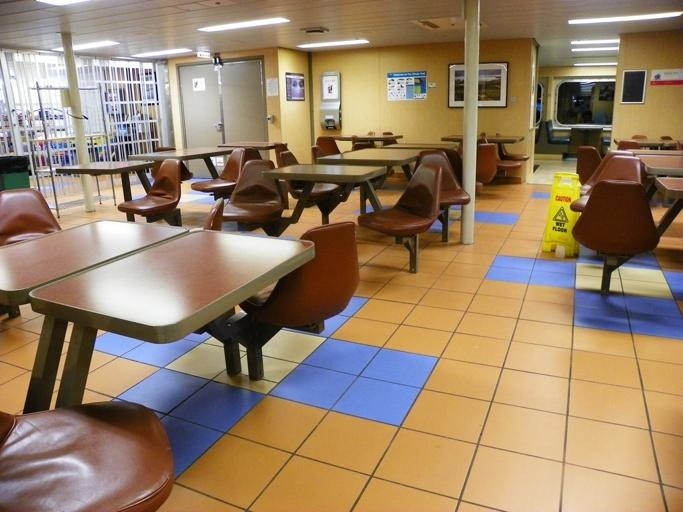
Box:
[447,61,508,108]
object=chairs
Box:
[495,133,529,185]
[571,145,644,213]
[279,152,342,226]
[119,160,184,228]
[632,134,647,144]
[617,142,642,150]
[231,222,362,383]
[273,145,292,167]
[351,142,375,152]
[367,132,373,138]
[413,150,471,244]
[602,138,610,145]
[0,401,176,512]
[535,119,546,146]
[660,136,671,139]
[359,165,444,275]
[191,149,247,200]
[223,161,292,238]
[477,144,499,186]
[312,146,323,164]
[544,120,571,145]
[571,181,659,297]
[1,189,63,248]
[316,137,342,157]
[153,148,195,182]
[383,131,399,145]
[190,197,244,377]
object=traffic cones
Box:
[539,171,580,257]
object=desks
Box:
[614,139,682,151]
[317,149,419,190]
[128,147,235,181]
[636,154,682,201]
[327,135,404,147]
[57,161,157,222]
[385,143,461,176]
[261,164,388,221]
[656,177,683,242]
[0,220,318,416]
[217,142,289,161]
[440,135,524,145]
[562,129,604,160]
[632,149,683,156]
